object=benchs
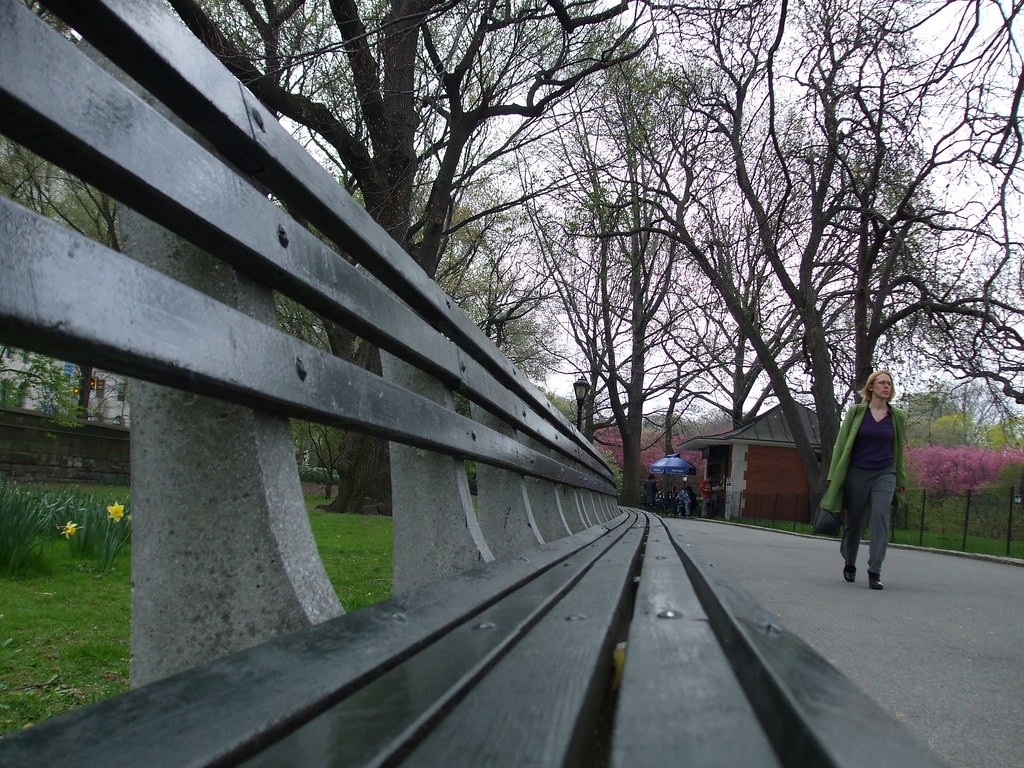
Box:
[1,0,946,768]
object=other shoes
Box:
[844,565,857,582]
[868,576,883,590]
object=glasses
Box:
[872,381,892,386]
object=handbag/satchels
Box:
[813,504,840,537]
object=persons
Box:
[669,484,694,517]
[699,475,716,518]
[818,370,909,590]
[644,473,658,509]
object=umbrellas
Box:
[650,453,695,476]
